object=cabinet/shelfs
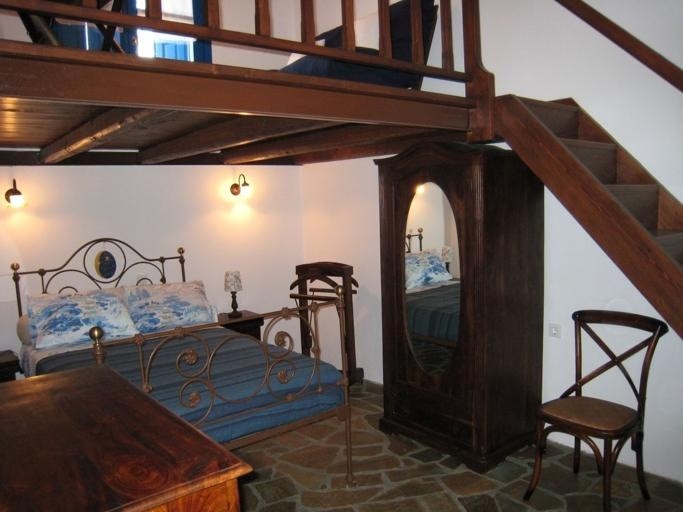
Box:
[373,137,546,475]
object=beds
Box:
[8,237,356,483]
[404,227,459,348]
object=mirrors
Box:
[400,178,465,378]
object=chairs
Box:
[522,309,669,512]
[279,0,438,95]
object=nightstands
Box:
[216,309,265,340]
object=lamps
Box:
[230,173,252,200]
[4,178,26,209]
[224,270,244,319]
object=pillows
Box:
[24,280,220,350]
[406,253,454,291]
